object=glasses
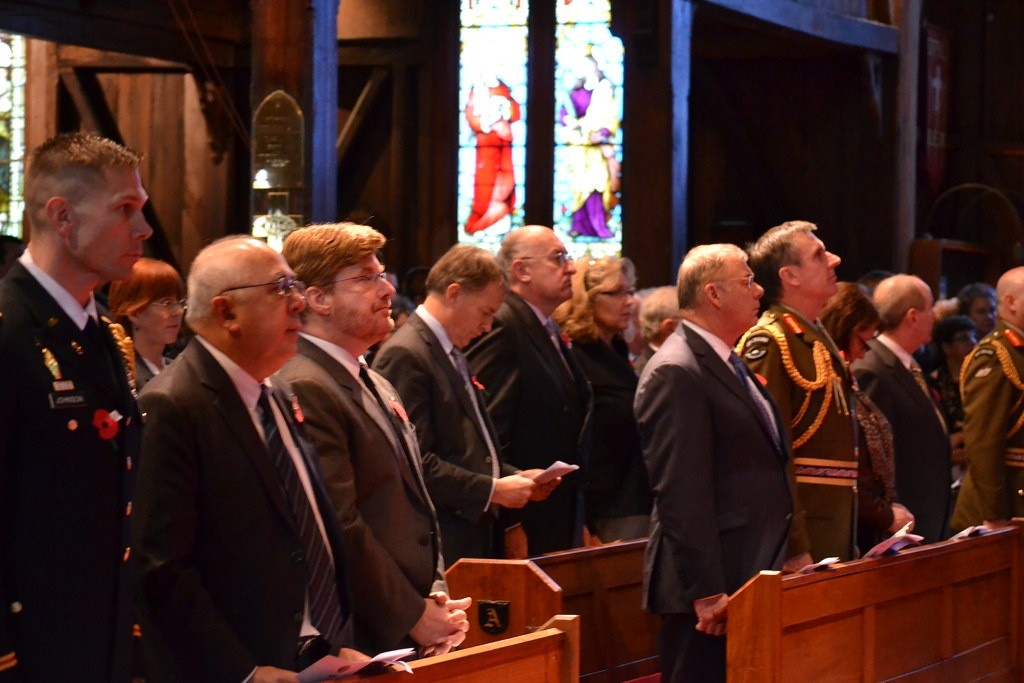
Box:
[513,252,571,266]
[319,270,386,287]
[218,275,302,295]
[856,333,872,353]
[601,286,636,298]
[151,299,188,309]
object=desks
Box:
[326,513,1024,683]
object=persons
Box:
[456,224,606,560]
[635,244,795,683]
[128,234,371,683]
[559,256,686,548]
[0,130,146,683]
[107,257,187,393]
[264,217,470,677]
[944,264,1024,535]
[735,219,1000,578]
[371,241,563,574]
[364,296,416,371]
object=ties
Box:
[911,361,947,437]
[450,346,499,480]
[359,365,439,572]
[728,350,780,452]
[81,314,120,392]
[546,320,573,381]
[258,385,343,644]
